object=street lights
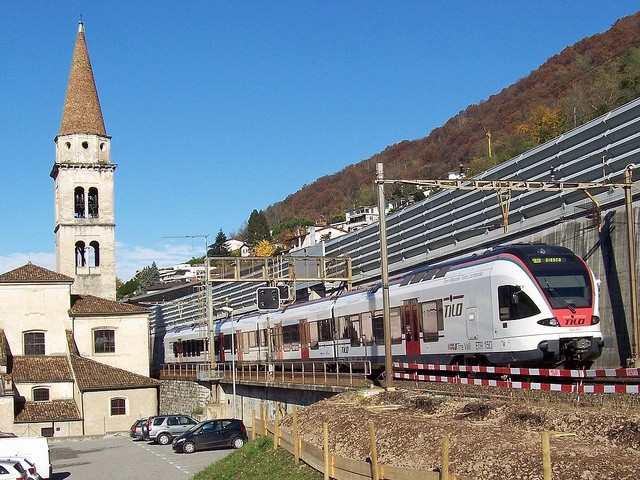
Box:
[215,307,237,418]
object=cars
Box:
[130,419,149,441]
[0,459,27,480]
[0,432,18,438]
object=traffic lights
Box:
[257,286,280,310]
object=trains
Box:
[150,243,604,375]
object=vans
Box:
[1,456,40,480]
[142,414,201,445]
[172,418,248,454]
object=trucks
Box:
[0,437,52,479]
